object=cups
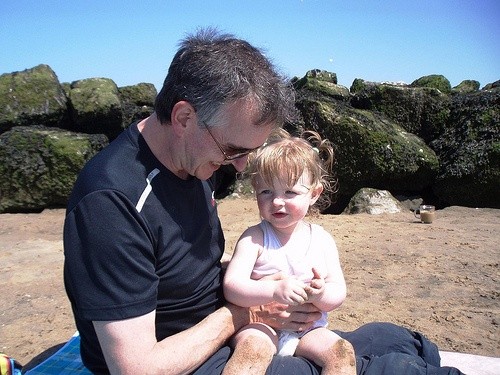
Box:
[414,205,435,224]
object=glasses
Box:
[201,115,265,160]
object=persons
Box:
[62,25,464,374]
[221,127,358,374]
